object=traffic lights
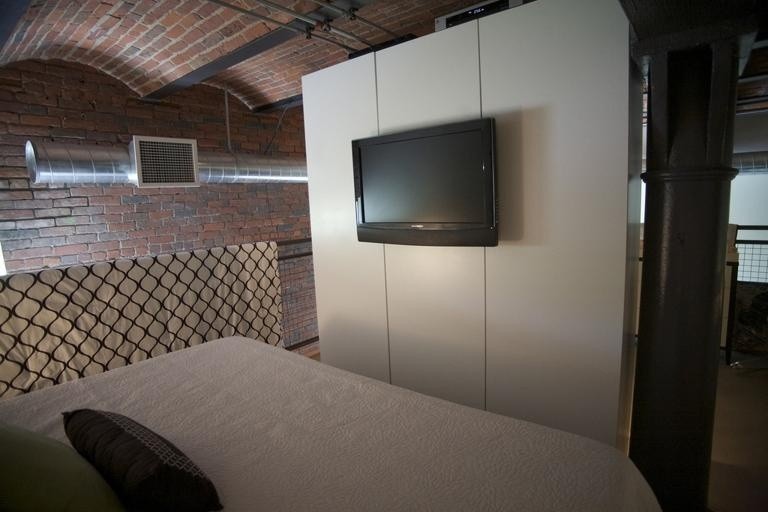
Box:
[60,407,220,510]
[1,415,116,510]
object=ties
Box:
[352,116,498,247]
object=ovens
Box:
[2,334,657,511]
